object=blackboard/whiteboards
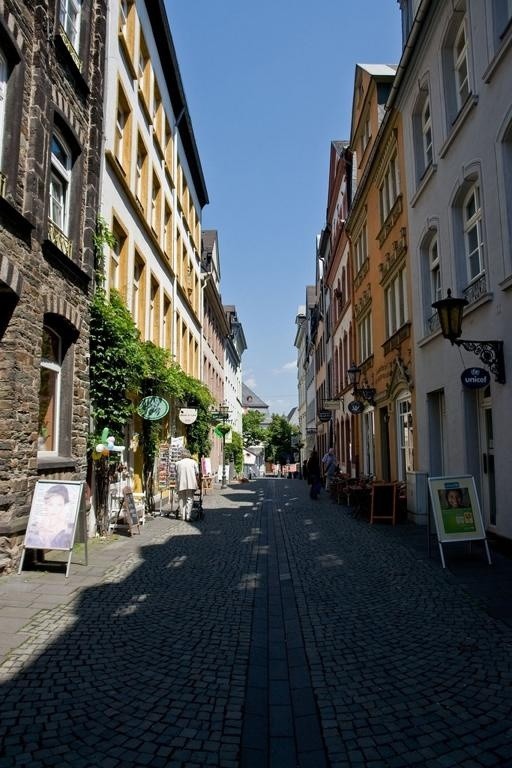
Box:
[124,492,140,529]
[371,482,396,518]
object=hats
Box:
[44,486,69,503]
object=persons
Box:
[27,485,73,549]
[445,488,466,509]
[276,447,336,501]
[174,449,200,522]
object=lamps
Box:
[430,287,506,385]
[346,361,376,408]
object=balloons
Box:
[93,427,125,460]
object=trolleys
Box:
[176,482,204,520]
